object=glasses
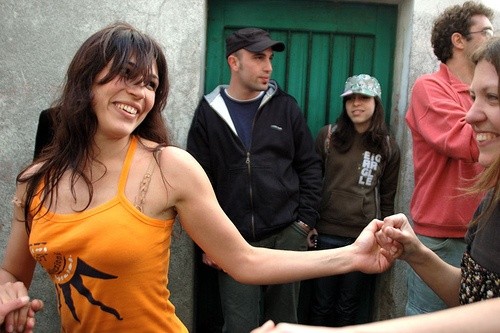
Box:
[470,30,494,39]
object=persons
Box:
[305,75,401,330]
[275,39,500,333]
[186,25,320,333]
[0,23,404,333]
[390,0,495,318]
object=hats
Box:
[225,26,285,57]
[339,73,382,100]
